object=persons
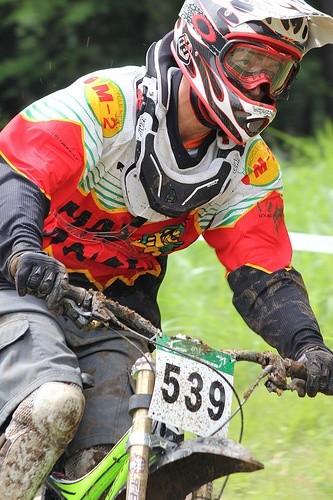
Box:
[0,0,333,500]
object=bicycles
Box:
[6,256,329,500]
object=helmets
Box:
[170,0,333,145]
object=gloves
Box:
[15,252,71,311]
[291,344,333,398]
